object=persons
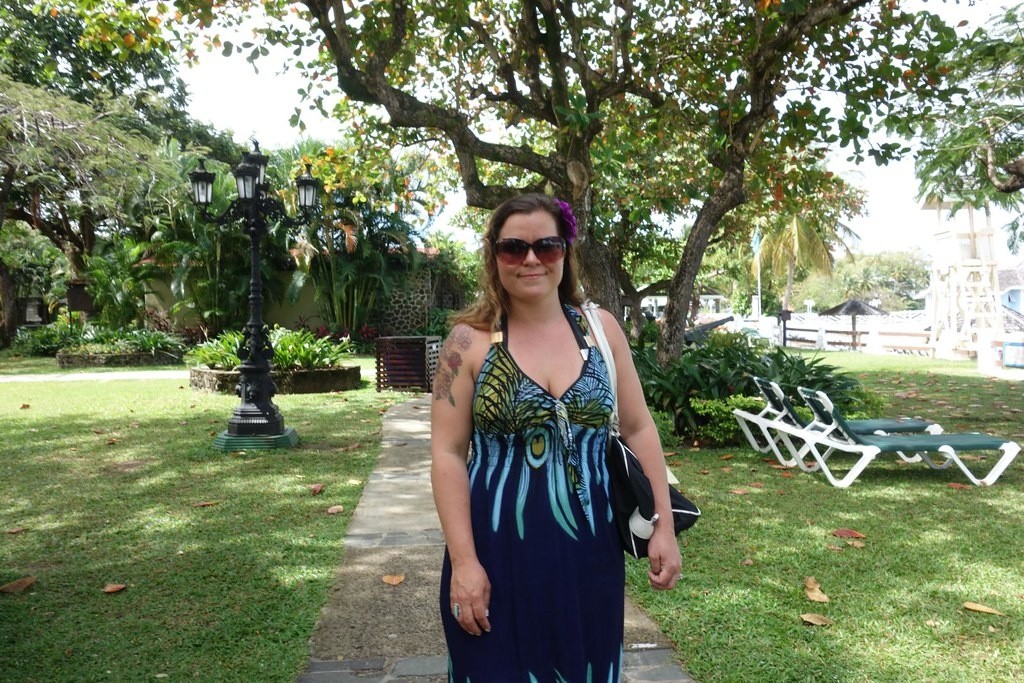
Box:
[431,193,681,683]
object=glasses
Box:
[489,235,567,265]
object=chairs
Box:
[770,386,1021,487]
[733,377,944,469]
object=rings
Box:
[452,602,460,617]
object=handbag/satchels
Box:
[607,434,702,559]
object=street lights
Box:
[185,140,318,455]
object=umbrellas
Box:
[817,301,890,352]
